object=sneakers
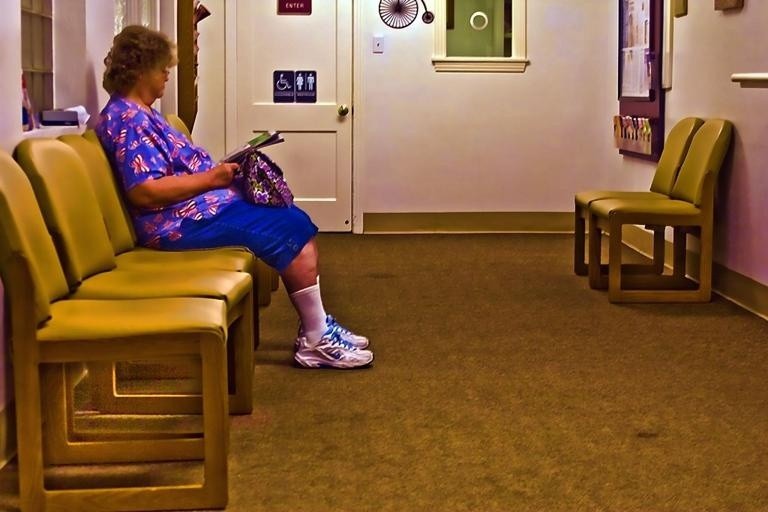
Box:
[295,328,374,368]
[295,314,369,350]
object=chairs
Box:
[15,136,255,442]
[2,152,231,512]
[55,133,256,393]
[572,116,734,305]
[167,112,280,308]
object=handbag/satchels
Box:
[243,152,293,207]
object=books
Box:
[220,128,285,163]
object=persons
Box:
[93,25,374,371]
